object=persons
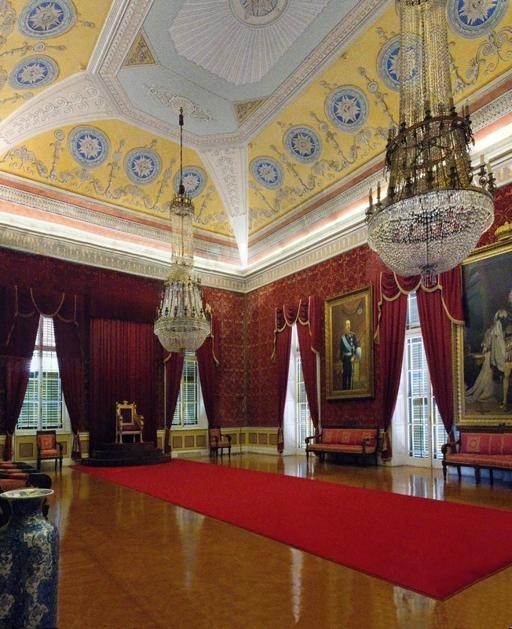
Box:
[338,318,362,391]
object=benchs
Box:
[305,424,380,469]
[441,431,512,486]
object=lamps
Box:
[366,1,496,293]
[153,105,212,355]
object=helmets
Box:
[355,347,361,358]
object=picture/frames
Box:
[323,285,378,401]
[452,237,512,431]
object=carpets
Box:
[67,456,512,602]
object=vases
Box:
[0,488,58,628]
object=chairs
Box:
[115,400,145,444]
[208,425,231,459]
[36,430,63,471]
